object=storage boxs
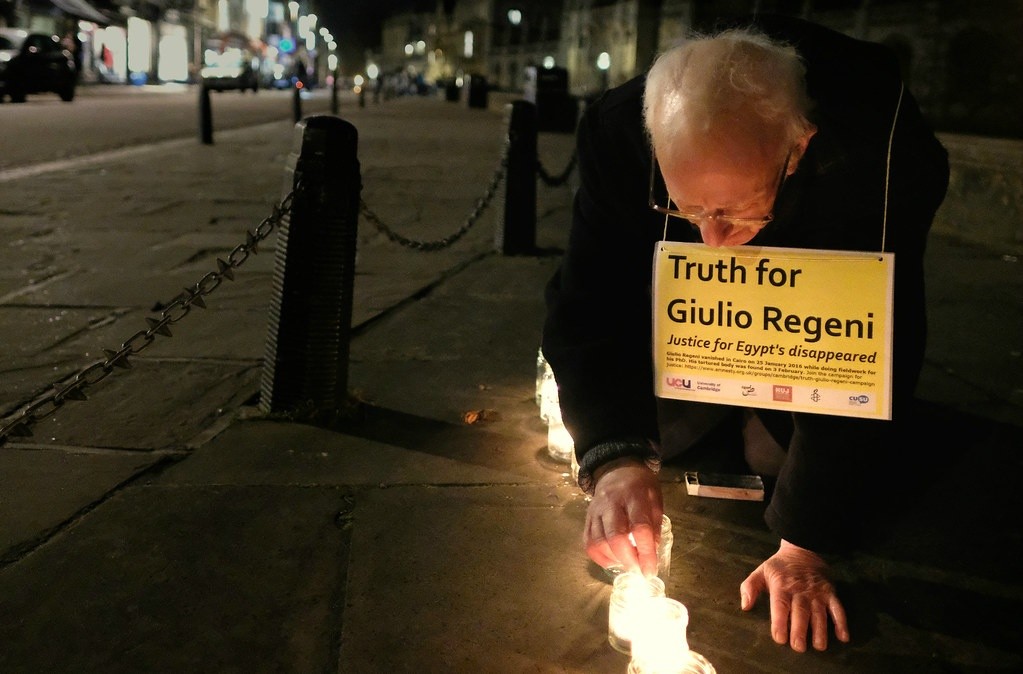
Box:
[685,472,765,501]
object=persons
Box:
[297,60,310,90]
[540,16,950,654]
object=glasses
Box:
[648,145,797,226]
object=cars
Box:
[204,59,258,93]
[0,27,81,104]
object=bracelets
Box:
[578,440,661,498]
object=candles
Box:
[535,350,716,674]
